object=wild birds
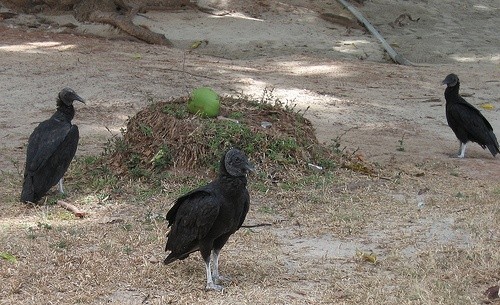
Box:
[19,87,86,204]
[163,148,258,292]
[438,72,500,160]
